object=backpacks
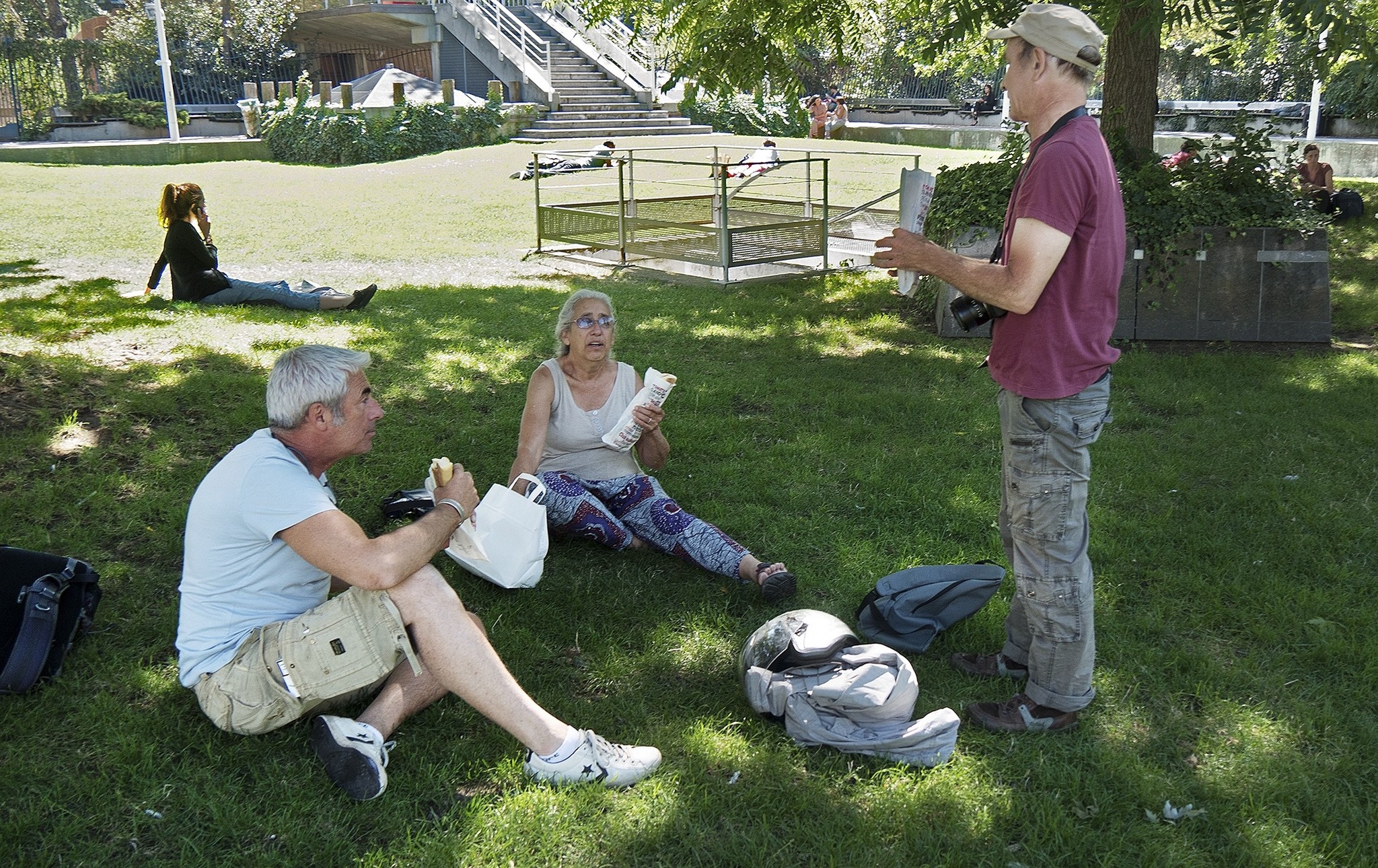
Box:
[854,559,1006,655]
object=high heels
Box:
[969,121,978,126]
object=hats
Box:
[986,3,1103,73]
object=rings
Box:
[648,417,653,422]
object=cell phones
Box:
[191,204,204,218]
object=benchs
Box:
[810,127,1031,152]
[1154,131,1378,177]
[1155,112,1303,133]
[866,107,1002,125]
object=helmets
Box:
[738,608,860,726]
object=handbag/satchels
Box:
[443,473,550,590]
[1327,187,1364,219]
[0,544,101,694]
[184,267,232,302]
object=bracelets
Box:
[437,498,465,528]
[204,238,213,240]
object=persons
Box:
[824,96,848,140]
[1160,140,1205,170]
[1284,144,1334,201]
[970,85,994,126]
[505,290,797,603]
[120,183,378,310]
[823,84,842,112]
[874,4,1127,731]
[806,94,828,138]
[510,141,629,181]
[718,141,783,178]
[174,344,663,801]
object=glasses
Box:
[569,315,614,328]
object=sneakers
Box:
[951,651,1030,681]
[966,689,1077,733]
[523,727,663,789]
[348,284,377,310]
[312,714,397,802]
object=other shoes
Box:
[510,172,521,179]
[520,171,527,181]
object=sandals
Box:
[755,561,796,600]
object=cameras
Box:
[949,292,1007,334]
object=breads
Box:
[431,457,452,486]
